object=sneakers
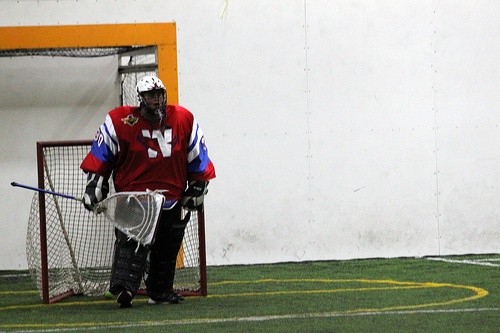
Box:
[147,294,183,304]
[115,289,133,309]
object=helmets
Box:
[136,75,169,121]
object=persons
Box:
[80,76,216,308]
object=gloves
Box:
[82,172,109,214]
[182,180,209,211]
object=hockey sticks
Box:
[10,181,167,248]
